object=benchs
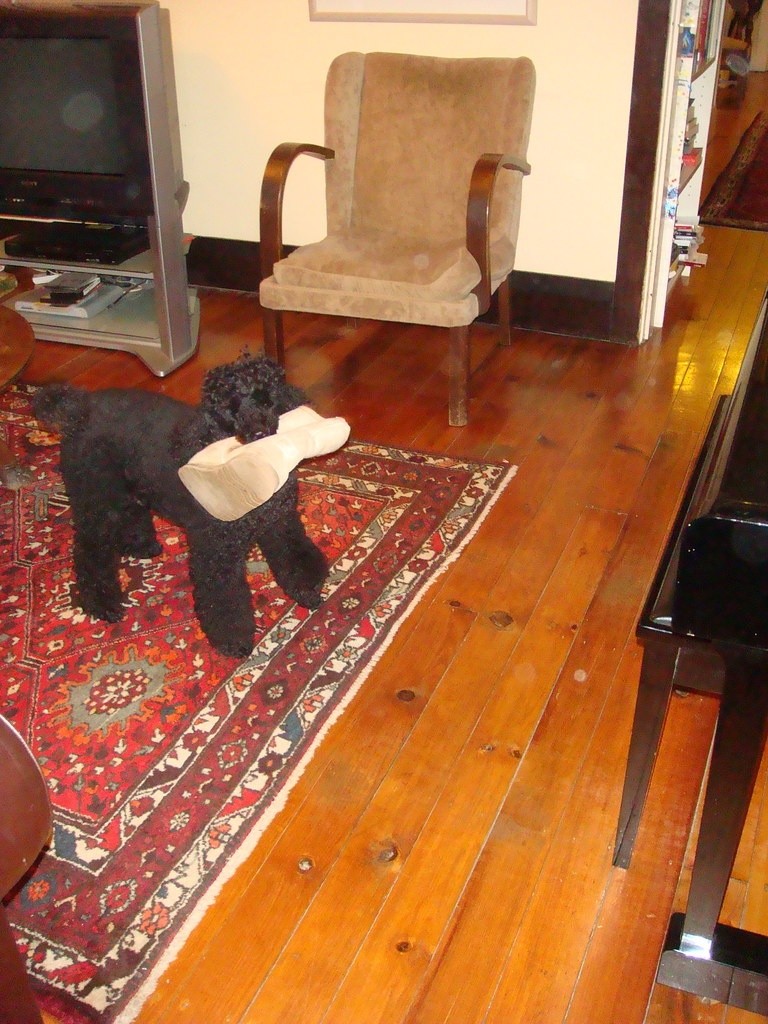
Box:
[614,392,730,870]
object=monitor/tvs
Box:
[0,10,153,267]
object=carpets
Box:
[698,109,768,233]
[1,375,517,1024]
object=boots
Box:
[178,405,351,522]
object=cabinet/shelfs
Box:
[0,179,203,378]
[666,1,727,296]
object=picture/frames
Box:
[310,0,539,25]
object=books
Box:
[678,98,703,190]
[669,224,708,287]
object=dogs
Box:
[29,347,330,660]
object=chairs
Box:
[257,51,540,427]
